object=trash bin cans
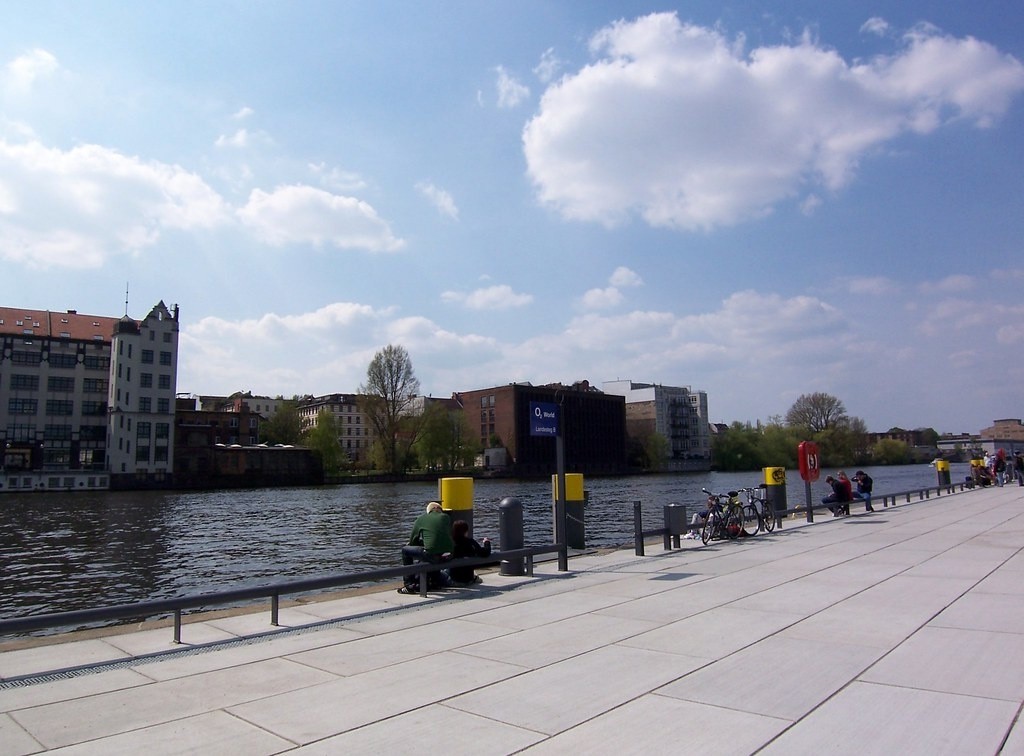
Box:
[1006,462,1013,482]
[498,496,525,577]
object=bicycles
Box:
[738,484,775,536]
[701,487,744,545]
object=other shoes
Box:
[832,506,846,517]
[684,533,697,539]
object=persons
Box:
[684,491,749,539]
[441,519,491,587]
[821,470,853,517]
[851,470,874,512]
[398,502,454,593]
[976,447,1024,488]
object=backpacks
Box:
[413,560,451,592]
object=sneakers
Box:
[397,586,416,594]
